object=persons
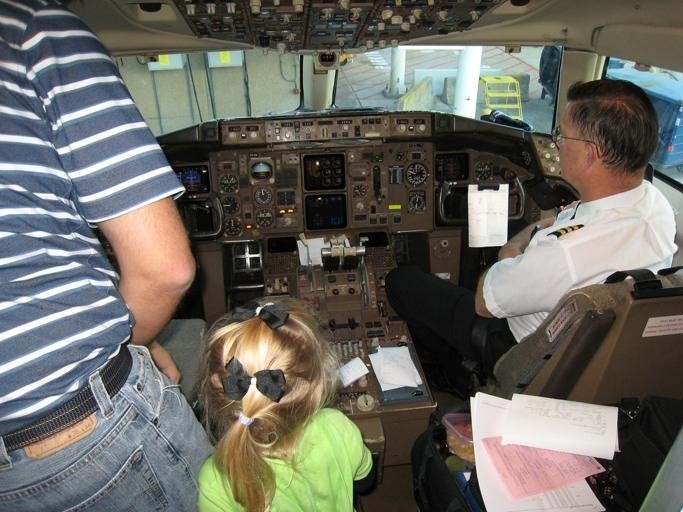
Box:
[385,78,678,400]
[198,295,375,512]
[0,0,218,512]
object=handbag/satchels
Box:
[411,411,488,512]
[601,394,682,510]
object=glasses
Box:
[551,126,599,159]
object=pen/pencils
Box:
[530,224,540,239]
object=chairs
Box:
[513,265,683,402]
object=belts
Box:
[2,345,132,451]
